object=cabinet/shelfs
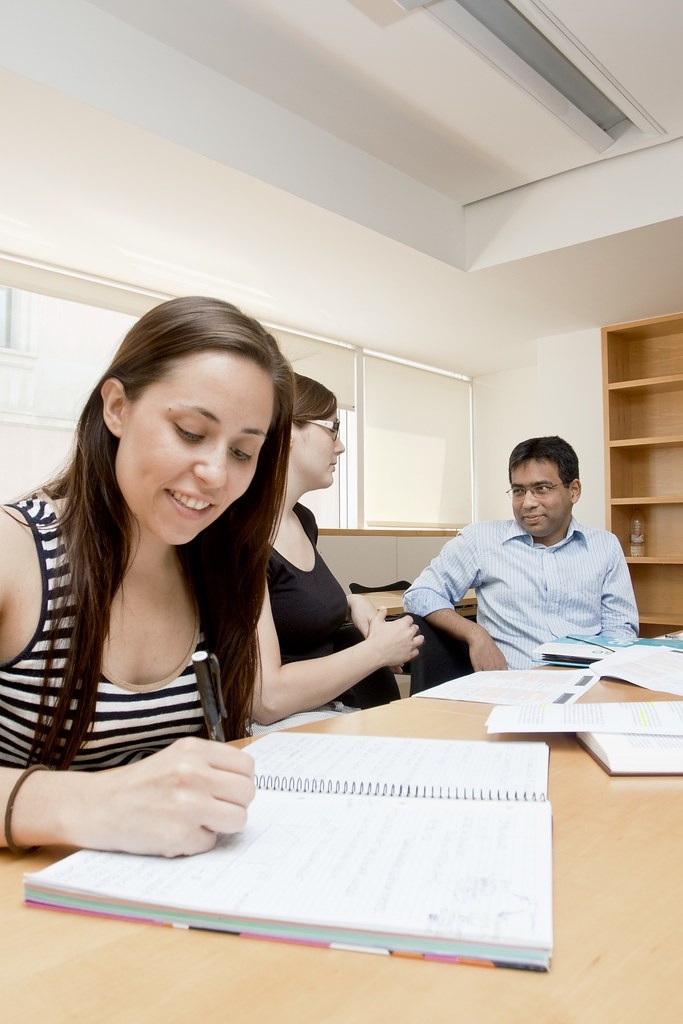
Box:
[601,312,683,638]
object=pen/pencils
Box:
[191,651,228,742]
[665,635,678,638]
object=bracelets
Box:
[4,764,49,857]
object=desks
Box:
[0,588,683,1024]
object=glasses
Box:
[306,419,340,442]
[505,482,564,500]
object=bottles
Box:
[630,508,645,557]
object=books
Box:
[20,730,554,975]
[573,728,683,777]
[529,629,683,672]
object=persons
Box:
[402,434,641,675]
[0,296,298,859]
[245,371,424,728]
